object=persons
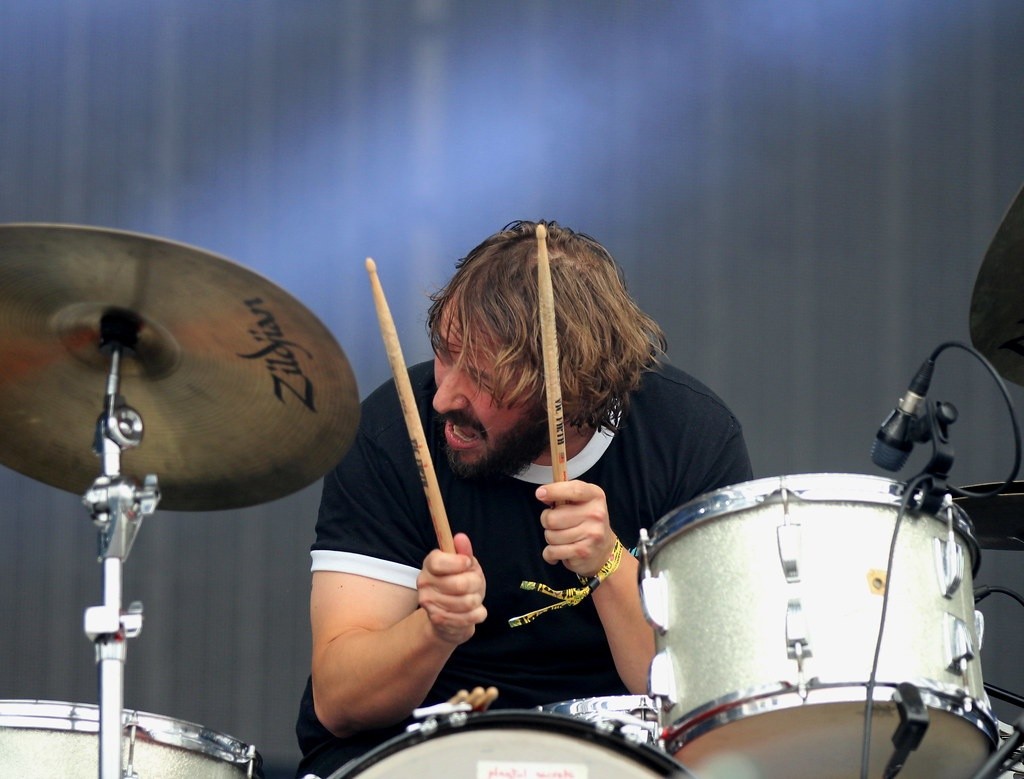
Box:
[293,219,754,779]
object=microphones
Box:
[870,359,936,471]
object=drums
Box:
[321,700,698,779]
[0,692,263,779]
[640,474,1005,779]
[534,690,681,754]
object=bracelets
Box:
[508,535,624,627]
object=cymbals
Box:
[948,479,1024,553]
[0,221,363,518]
